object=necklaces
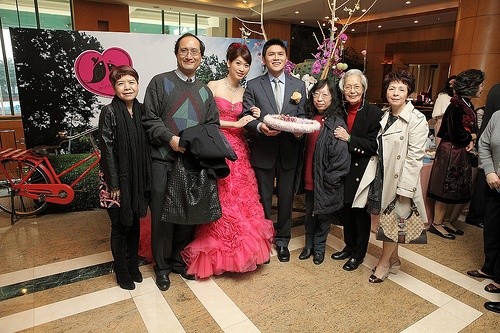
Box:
[224,79,239,93]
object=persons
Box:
[142,33,237,291]
[139,43,275,279]
[333,67,384,270]
[238,39,308,265]
[352,68,429,283]
[293,78,350,265]
[96,65,145,291]
[431,69,500,313]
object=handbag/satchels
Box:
[442,143,473,193]
[160,152,222,226]
[98,169,122,208]
[375,195,428,244]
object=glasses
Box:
[311,92,332,99]
[179,48,201,56]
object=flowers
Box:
[285,34,348,76]
[290,90,302,104]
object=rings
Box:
[296,136,299,140]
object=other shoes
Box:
[467,269,500,280]
[429,223,456,239]
[484,282,500,293]
[441,223,464,235]
[465,220,484,228]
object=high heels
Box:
[114,270,135,290]
[368,259,402,283]
[126,255,143,282]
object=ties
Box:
[272,78,281,113]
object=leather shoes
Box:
[342,257,363,271]
[484,301,500,313]
[314,251,326,264]
[156,274,170,291]
[172,268,195,280]
[276,246,290,261]
[331,250,351,260]
[299,248,314,259]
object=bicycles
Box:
[0,125,102,226]
